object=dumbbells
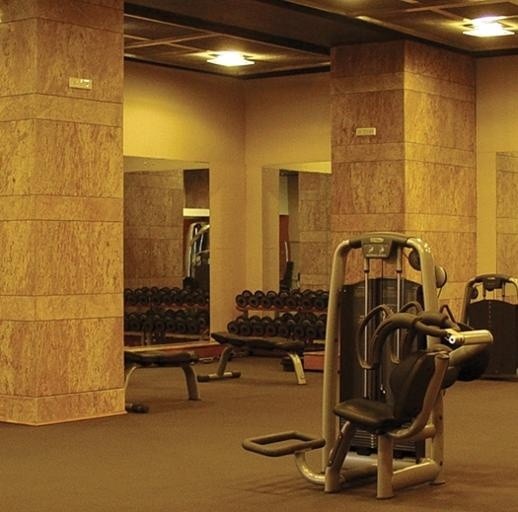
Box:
[122,285,210,335]
[226,289,329,340]
